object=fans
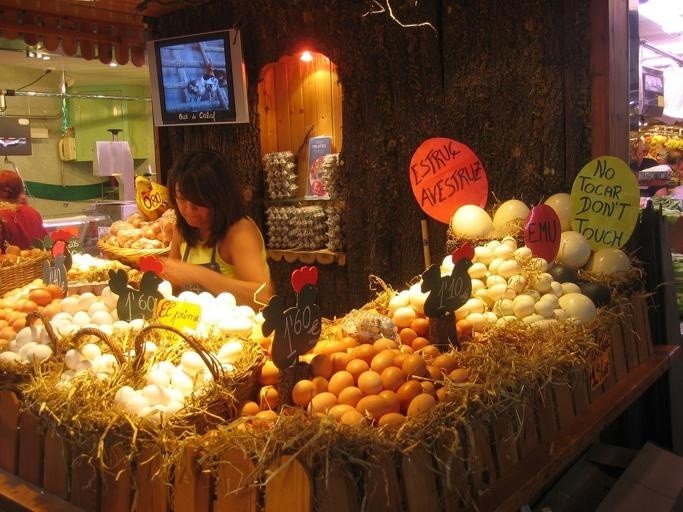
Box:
[15,41,64,57]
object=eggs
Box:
[0,238,581,433]
[101,208,176,248]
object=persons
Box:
[156,148,275,314]
[0,170,48,254]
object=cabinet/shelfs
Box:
[64,84,147,162]
[37,209,119,259]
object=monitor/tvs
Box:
[145,28,251,128]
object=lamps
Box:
[27,45,51,60]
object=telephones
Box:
[59,137,76,162]
